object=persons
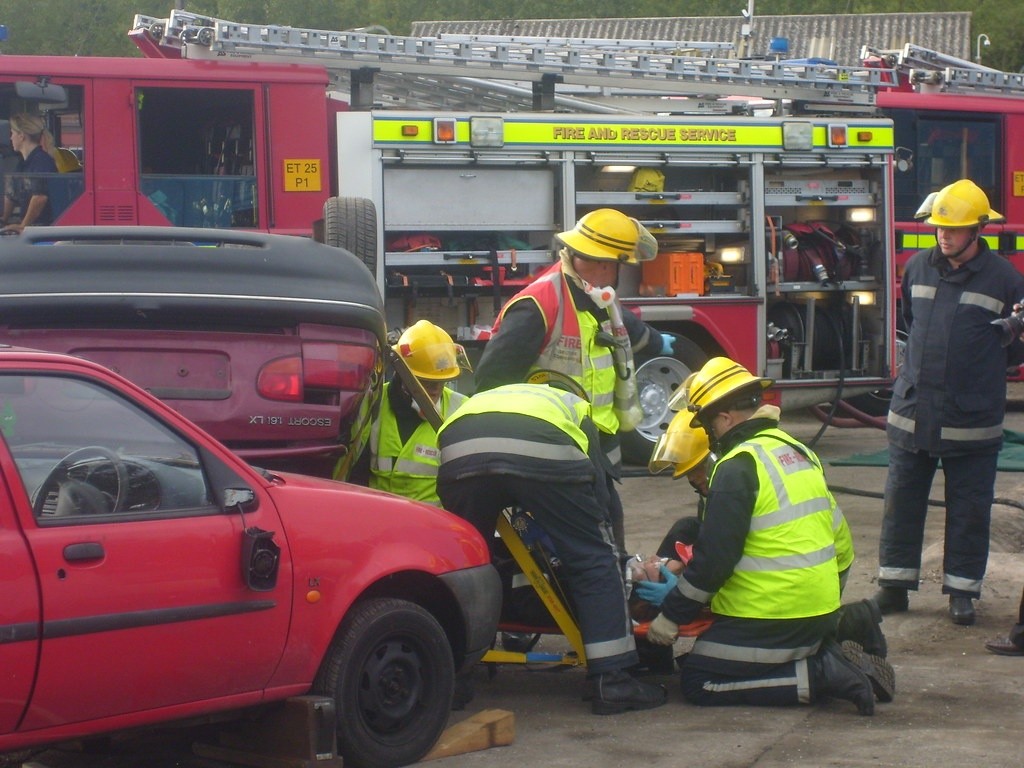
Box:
[635,355,895,715]
[865,178,1024,623]
[473,208,676,565]
[335,319,467,511]
[0,112,83,230]
[435,367,676,714]
[482,536,693,626]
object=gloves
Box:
[649,610,680,647]
[634,564,680,607]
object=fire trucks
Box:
[0,9,1024,470]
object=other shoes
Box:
[837,640,895,701]
[591,668,671,715]
[948,593,975,625]
[501,631,532,649]
[871,586,909,614]
[812,636,874,717]
[841,597,887,658]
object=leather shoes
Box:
[984,634,1024,656]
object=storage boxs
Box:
[644,252,707,296]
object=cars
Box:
[0,220,392,484]
[0,348,508,768]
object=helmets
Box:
[687,357,775,428]
[555,208,641,266]
[391,320,461,381]
[525,369,591,403]
[924,179,1004,229]
[665,408,711,478]
